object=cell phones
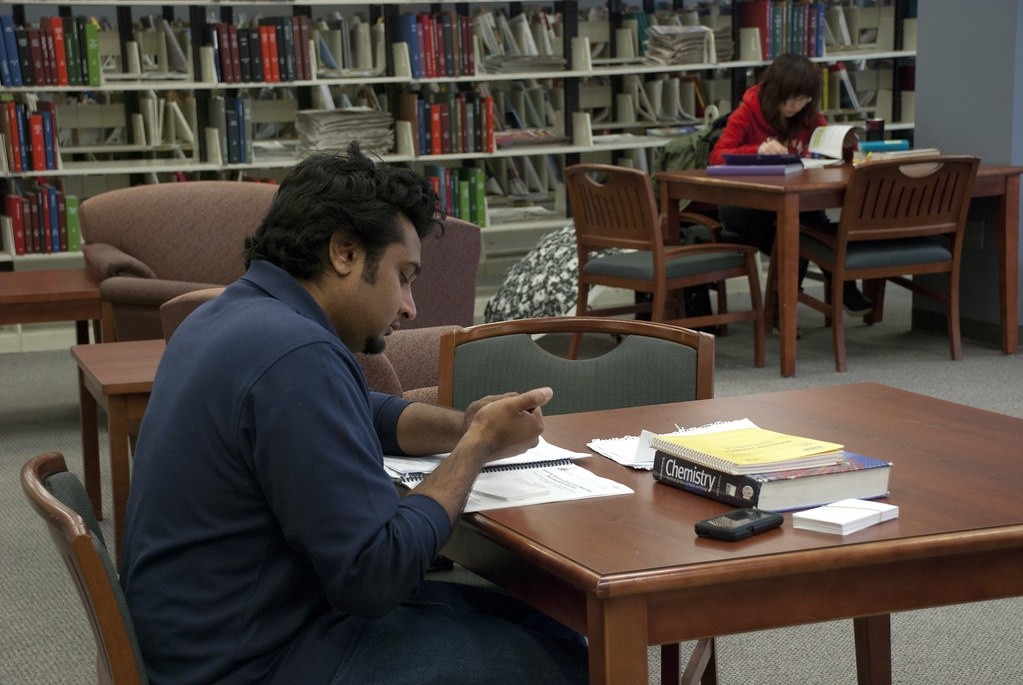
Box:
[694,508,784,541]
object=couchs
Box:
[97,277,220,339]
[77,182,277,341]
[398,216,483,330]
[161,286,460,406]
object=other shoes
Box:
[844,288,878,311]
[776,321,802,339]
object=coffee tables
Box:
[71,339,167,567]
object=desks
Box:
[385,382,1023,685]
[651,164,1023,379]
[0,269,98,346]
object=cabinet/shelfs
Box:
[0,0,919,356]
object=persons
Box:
[115,154,589,685]
[708,54,874,337]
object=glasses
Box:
[787,96,811,104]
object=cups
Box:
[866,118,884,142]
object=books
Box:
[0,0,860,256]
[385,445,635,512]
[648,427,894,512]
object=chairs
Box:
[19,452,148,685]
[439,318,719,685]
[765,154,981,373]
[564,164,765,368]
